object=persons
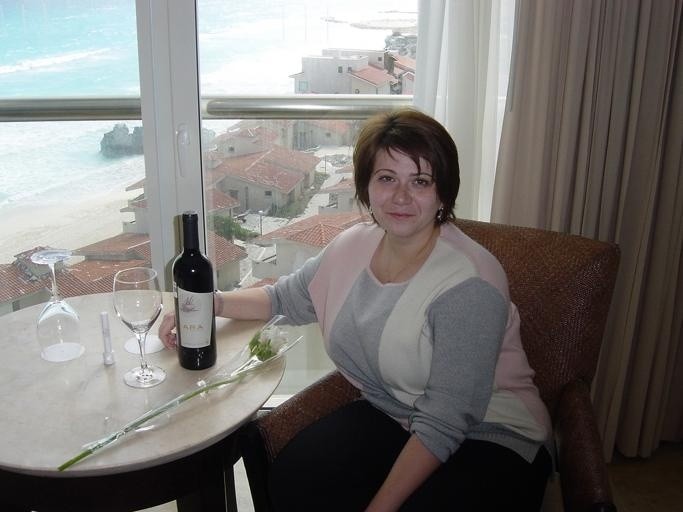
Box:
[159,108,553,512]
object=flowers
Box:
[57,313,306,471]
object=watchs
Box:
[213,289,224,318]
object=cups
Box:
[111,266,166,389]
[28,249,83,363]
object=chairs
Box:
[262,216,620,510]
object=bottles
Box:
[173,212,216,370]
